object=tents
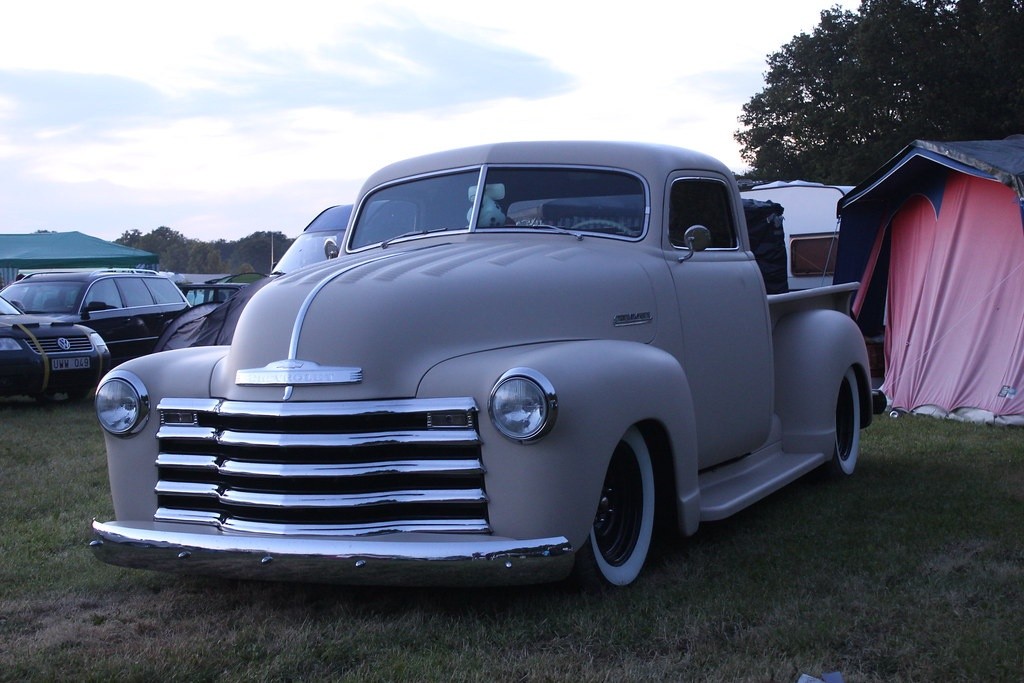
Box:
[0,231,159,291]
[833,134,1024,426]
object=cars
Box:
[0,295,112,399]
[176,281,249,307]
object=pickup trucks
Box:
[89,143,890,598]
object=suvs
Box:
[0,268,193,367]
